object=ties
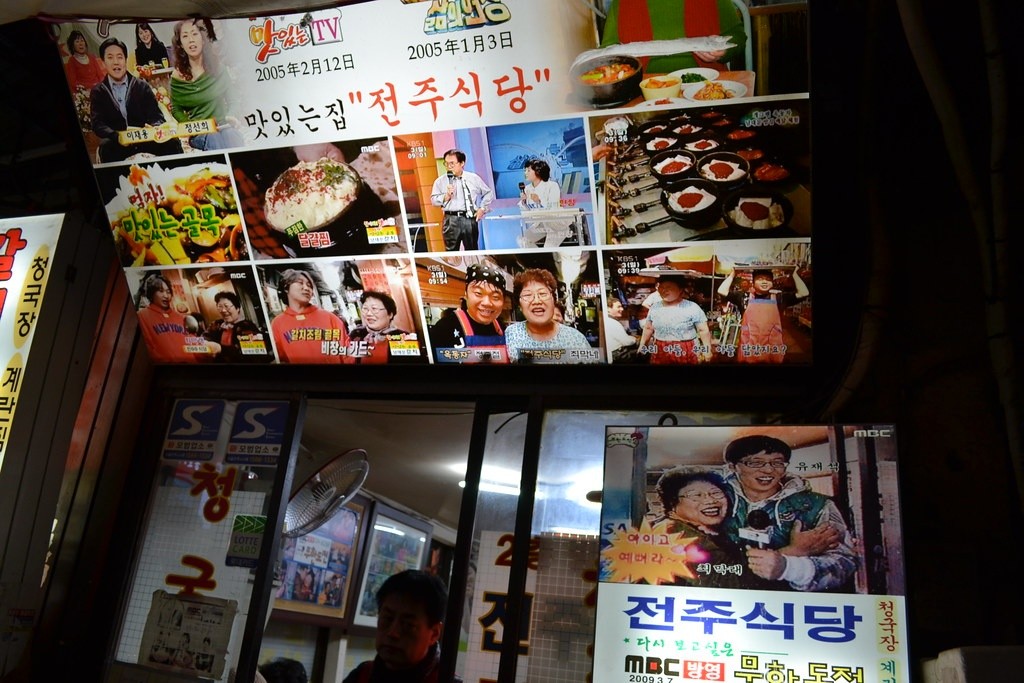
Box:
[458,176,475,218]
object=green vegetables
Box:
[287,158,359,194]
[681,72,708,83]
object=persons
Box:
[723,435,858,595]
[64,30,105,93]
[606,260,712,364]
[599,0,746,72]
[504,269,597,363]
[638,464,838,590]
[516,160,569,248]
[342,570,467,683]
[717,263,810,363]
[90,37,185,163]
[136,275,205,363]
[428,263,509,363]
[200,291,275,364]
[170,19,244,151]
[229,142,386,258]
[270,269,355,364]
[350,291,419,366]
[134,22,171,68]
[431,149,494,252]
[272,511,352,607]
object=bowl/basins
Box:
[626,106,796,241]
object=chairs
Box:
[724,0,752,71]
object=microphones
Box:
[736,509,773,552]
[517,181,527,206]
[446,169,454,193]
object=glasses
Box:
[442,161,459,168]
[518,286,554,303]
[678,490,726,499]
[216,303,234,313]
[359,304,387,316]
[738,460,789,469]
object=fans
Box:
[283,446,370,539]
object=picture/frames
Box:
[343,502,436,639]
[268,502,372,632]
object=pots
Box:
[264,159,387,258]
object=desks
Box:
[475,210,593,246]
[408,222,440,253]
[613,71,756,109]
[151,65,174,75]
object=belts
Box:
[445,210,467,217]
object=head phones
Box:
[138,272,174,298]
